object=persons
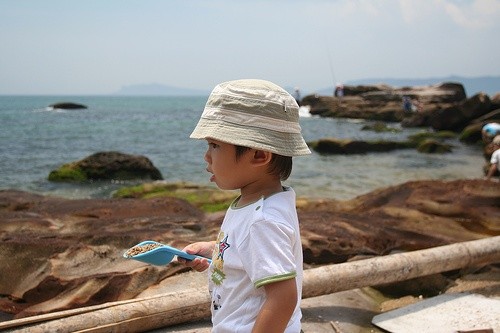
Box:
[482,122,500,182]
[334,84,343,97]
[177,78,311,333]
[399,95,424,116]
[293,87,301,106]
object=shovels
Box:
[122,240,212,269]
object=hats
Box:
[189,79,312,156]
[482,123,500,137]
